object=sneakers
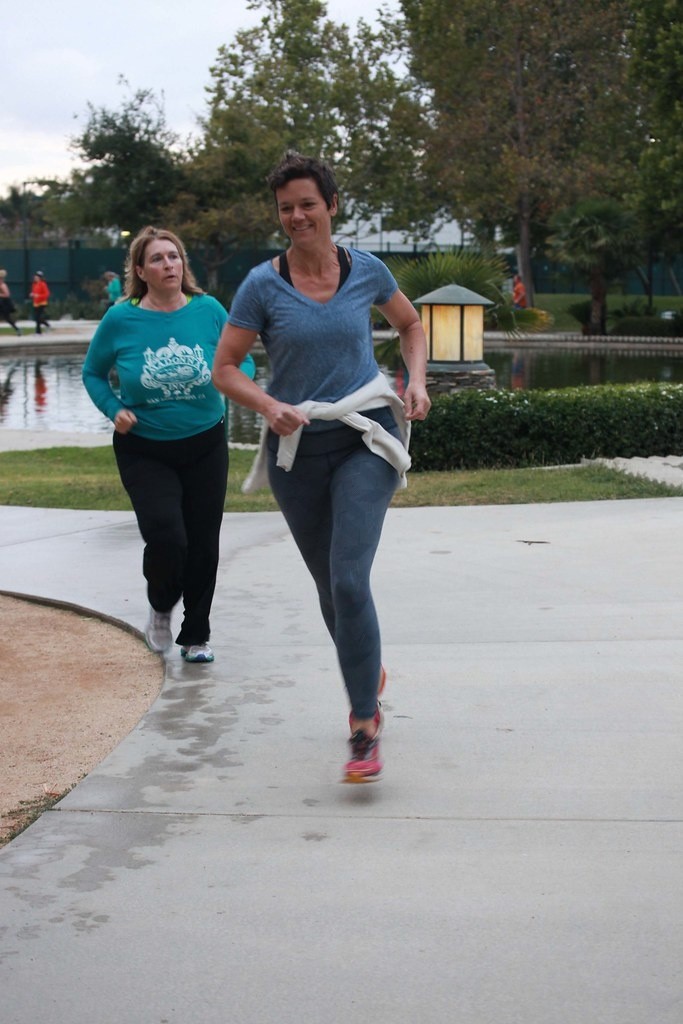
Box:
[180,644,214,661]
[338,702,385,783]
[144,605,172,653]
[377,665,385,696]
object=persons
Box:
[81,225,256,662]
[512,275,528,309]
[29,270,52,335]
[0,269,22,336]
[212,149,432,785]
[103,271,123,310]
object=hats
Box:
[34,270,44,277]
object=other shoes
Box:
[46,325,51,332]
[34,333,42,337]
[16,328,21,337]
